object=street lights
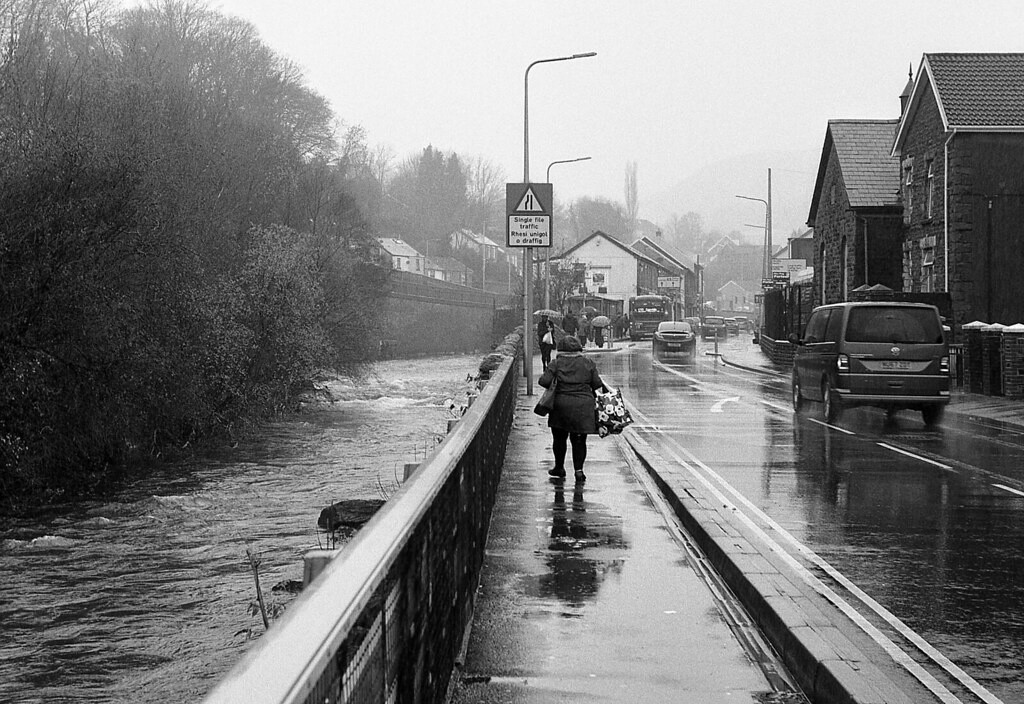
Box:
[523,51,598,392]
[734,194,768,340]
[544,155,594,310]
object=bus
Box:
[628,295,674,340]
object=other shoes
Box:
[574,470,587,481]
[547,466,567,478]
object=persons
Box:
[537,315,554,371]
[562,310,579,337]
[538,335,602,479]
[616,313,629,338]
[578,315,608,348]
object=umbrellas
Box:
[533,310,561,318]
[591,316,611,327]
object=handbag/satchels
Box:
[552,336,558,350]
[542,331,553,345]
[593,376,635,438]
[533,376,556,416]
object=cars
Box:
[653,320,697,358]
[683,315,750,339]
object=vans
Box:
[788,302,951,425]
